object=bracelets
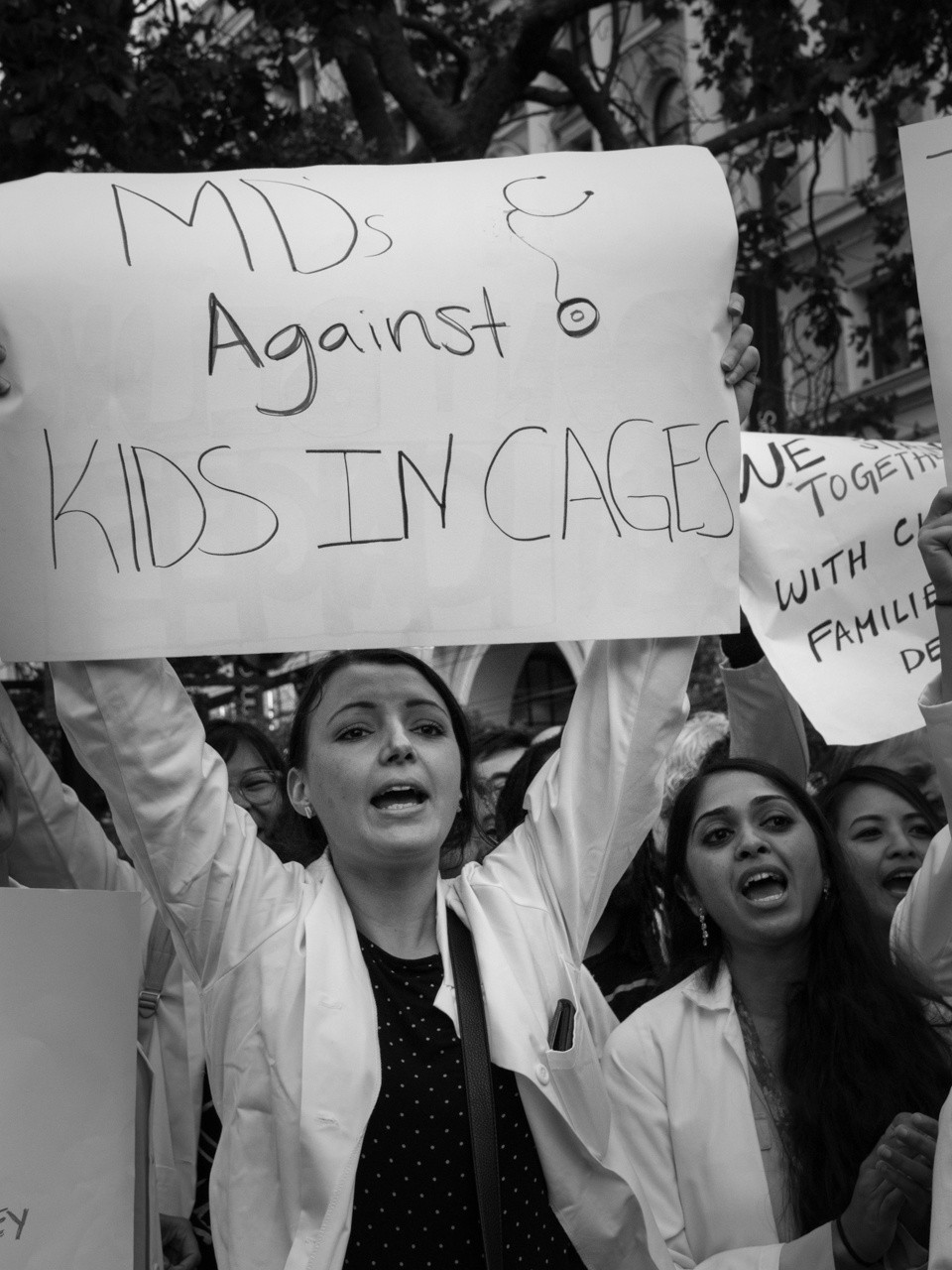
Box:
[836,1214,876,1270]
[934,600,952,606]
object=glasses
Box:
[226,770,285,806]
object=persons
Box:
[48,636,703,1270]
[438,482,952,1270]
[0,678,287,1270]
